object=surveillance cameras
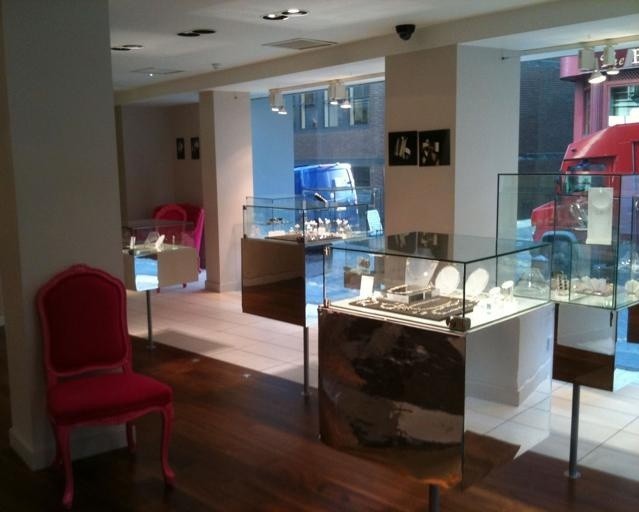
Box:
[395,23,416,40]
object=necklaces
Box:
[355,255,474,321]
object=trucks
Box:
[528,121,639,280]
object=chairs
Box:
[151,203,205,274]
[34,265,177,510]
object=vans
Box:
[294,160,361,232]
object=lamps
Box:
[328,79,351,109]
[577,39,619,84]
[269,88,288,115]
